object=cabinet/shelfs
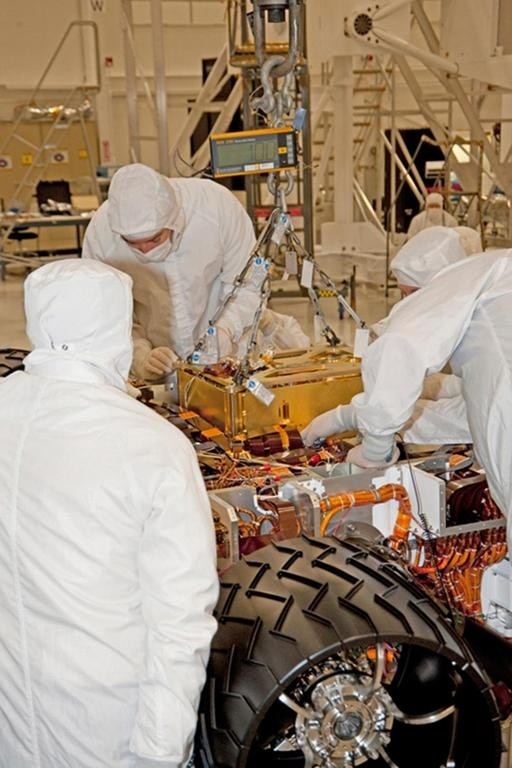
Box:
[0,119,100,257]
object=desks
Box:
[0,213,91,281]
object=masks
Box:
[127,241,173,264]
[427,208,442,220]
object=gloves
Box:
[301,402,346,448]
[134,341,178,381]
[346,444,400,469]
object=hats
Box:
[21,258,133,390]
[424,192,444,211]
[107,163,186,244]
[390,225,483,291]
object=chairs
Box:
[7,208,38,258]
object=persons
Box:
[300,191,511,640]
[82,164,267,383]
[256,309,310,357]
[1,260,220,767]
